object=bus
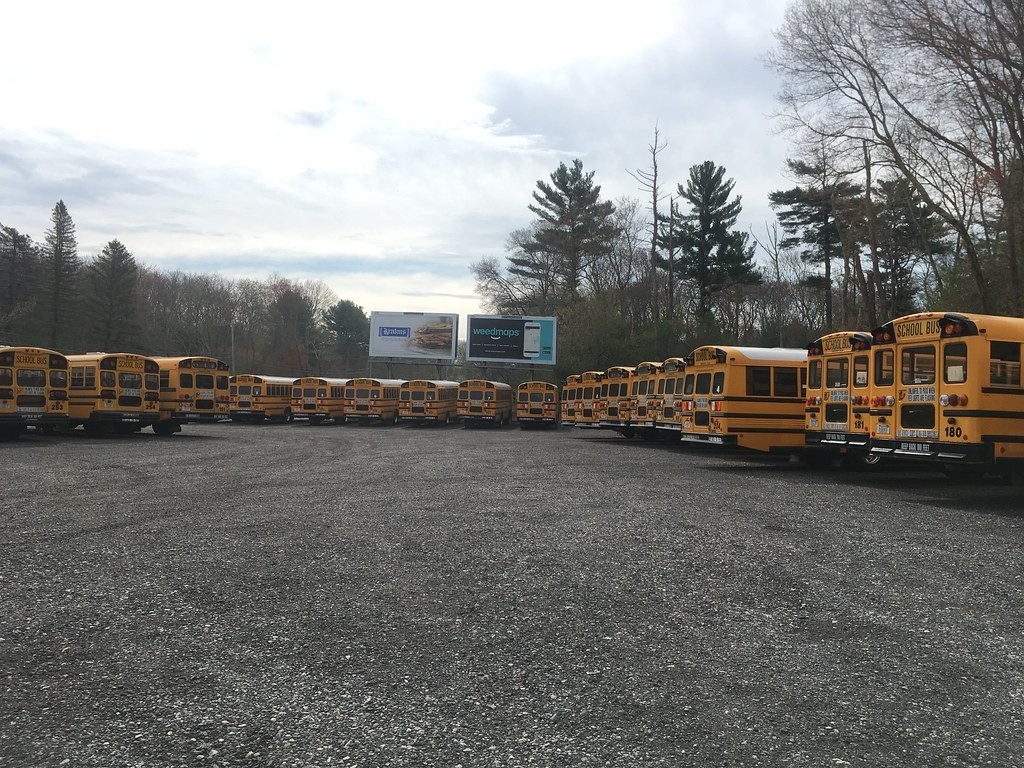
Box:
[560,312,1024,473]
[1,345,557,436]
